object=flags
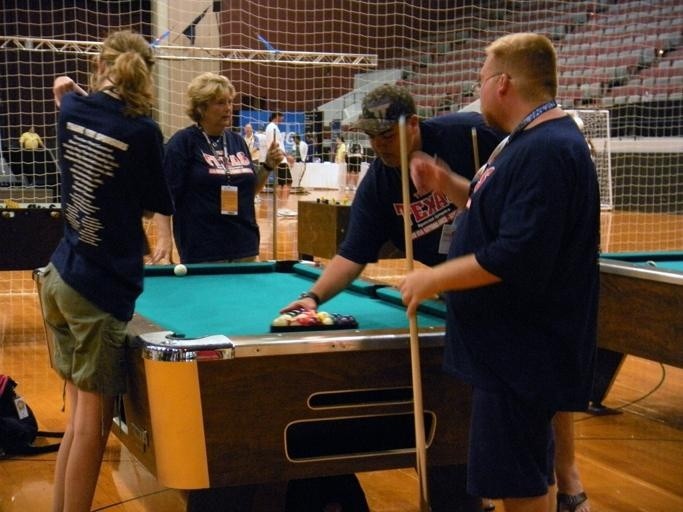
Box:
[149,23,178,49]
[182,5,210,45]
[255,32,281,63]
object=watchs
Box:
[298,288,320,304]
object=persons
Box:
[278,84,588,511]
[17,125,46,185]
[144,68,285,267]
[333,134,348,163]
[38,29,175,511]
[398,31,600,512]
[264,111,298,218]
[290,134,308,163]
[344,133,363,191]
[241,123,260,203]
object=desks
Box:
[41,258,471,511]
[292,161,374,261]
[593,251,682,370]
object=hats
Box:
[349,84,416,133]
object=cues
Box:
[472,126,480,174]
[398,117,429,511]
[273,129,276,261]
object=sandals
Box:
[556,489,587,512]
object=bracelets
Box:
[260,162,273,171]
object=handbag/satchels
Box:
[0,375,39,458]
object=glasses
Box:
[472,73,512,94]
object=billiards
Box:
[272,308,355,326]
[174,264,187,276]
